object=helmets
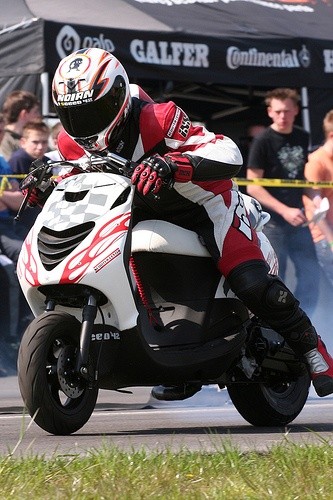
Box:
[52,47,130,152]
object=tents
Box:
[0,0,333,149]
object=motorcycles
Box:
[12,150,312,436]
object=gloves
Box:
[131,152,194,197]
[22,178,41,207]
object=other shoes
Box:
[304,339,333,397]
[152,385,200,401]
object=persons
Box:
[0,92,64,361]
[247,89,317,317]
[22,48,333,397]
[304,111,333,286]
